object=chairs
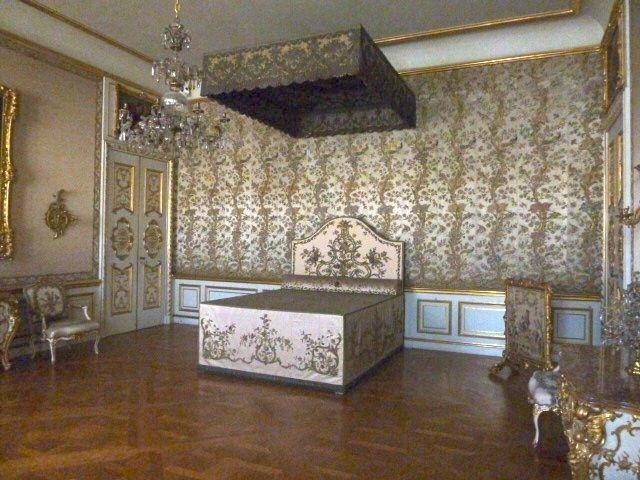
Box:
[24,280,100,364]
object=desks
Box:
[557,342,640,479]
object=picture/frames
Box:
[0,85,15,260]
[607,136,622,206]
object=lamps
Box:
[114,0,229,159]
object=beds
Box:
[198,217,406,395]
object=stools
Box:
[530,369,561,447]
